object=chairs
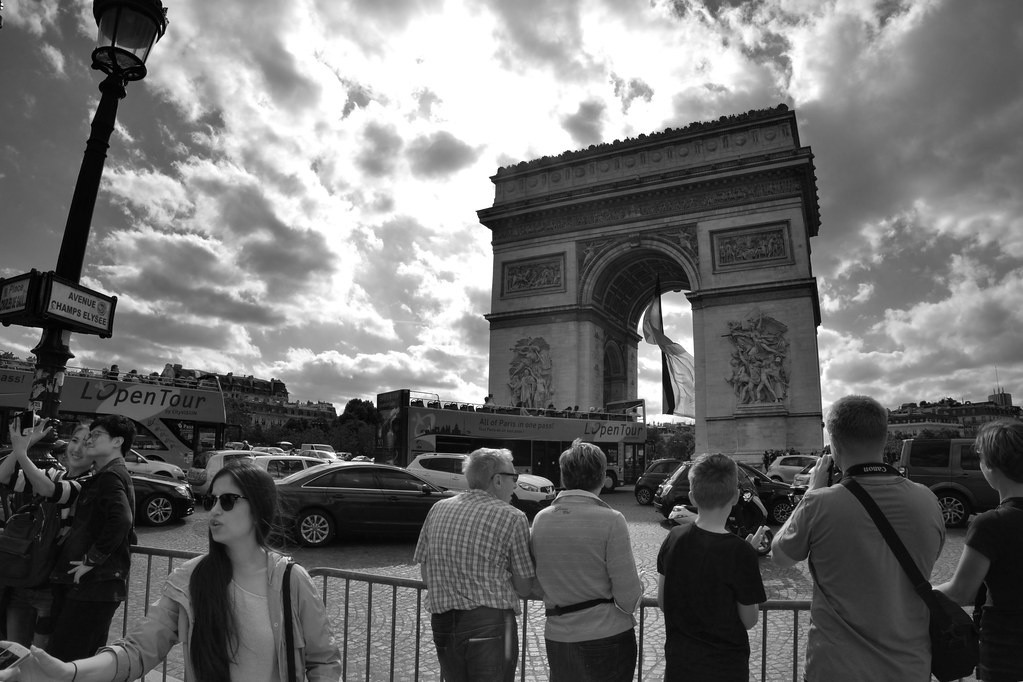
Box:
[291,462,303,474]
[359,478,373,488]
[281,462,291,475]
[0,356,201,387]
[411,398,634,422]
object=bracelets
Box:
[66,659,78,681]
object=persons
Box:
[659,227,699,262]
[508,261,561,289]
[771,394,947,682]
[504,344,551,408]
[0,409,138,662]
[413,447,535,682]
[0,463,344,682]
[725,311,786,406]
[532,436,646,682]
[655,452,768,682]
[720,231,786,265]
[580,238,611,272]
[933,419,1023,682]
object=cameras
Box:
[13,410,41,436]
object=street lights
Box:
[18,0,170,467]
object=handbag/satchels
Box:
[928,589,980,682]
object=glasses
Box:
[88,430,113,439]
[491,472,519,483]
[976,449,990,460]
[203,494,249,512]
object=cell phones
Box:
[0,642,32,672]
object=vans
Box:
[898,438,1000,528]
[188,441,345,503]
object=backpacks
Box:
[0,474,91,588]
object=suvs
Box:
[406,453,556,517]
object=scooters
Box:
[667,487,774,556]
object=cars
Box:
[634,455,842,523]
[336,451,371,462]
[82,448,196,528]
[272,463,461,547]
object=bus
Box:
[0,356,245,499]
[375,388,656,495]
